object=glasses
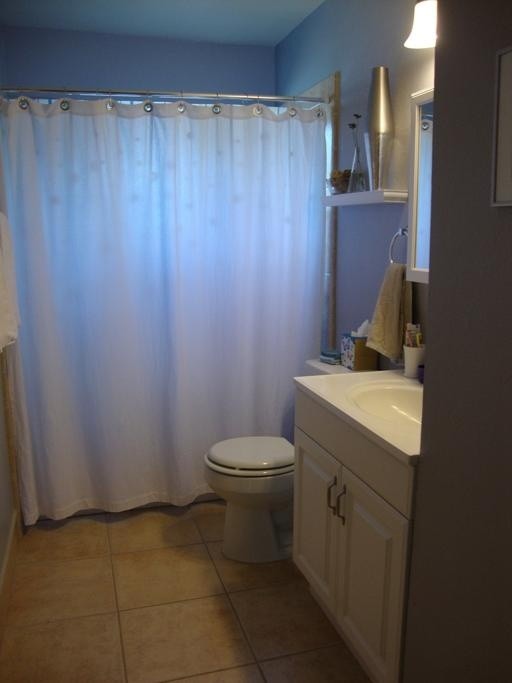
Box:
[400,0,442,53]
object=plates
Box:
[291,388,416,681]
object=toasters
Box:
[415,331,424,347]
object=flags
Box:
[363,58,396,197]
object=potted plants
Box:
[485,43,512,213]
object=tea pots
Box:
[411,95,435,275]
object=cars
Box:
[403,343,424,380]
